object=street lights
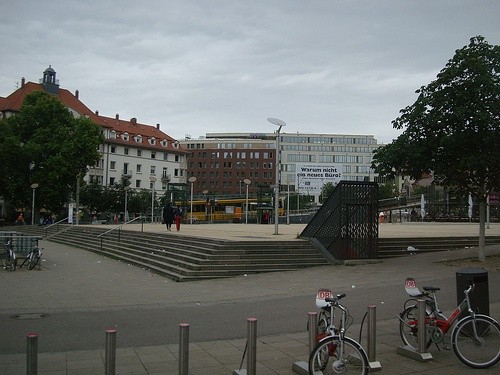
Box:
[149,176,156,224]
[188,177,196,223]
[30,183,39,225]
[267,117,287,235]
[244,178,252,224]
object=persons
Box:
[40,214,56,225]
[114,212,118,224]
[262,212,268,224]
[411,209,415,222]
[18,213,22,225]
[174,205,183,232]
[93,216,97,224]
[379,212,383,223]
[163,203,174,231]
[107,214,110,225]
[78,210,83,223]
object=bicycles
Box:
[19,244,42,269]
[398,275,499,368]
[3,240,17,270]
[305,294,369,375]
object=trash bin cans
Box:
[455,267,489,337]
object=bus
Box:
[171,199,284,223]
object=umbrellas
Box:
[421,194,425,222]
[468,192,473,222]
